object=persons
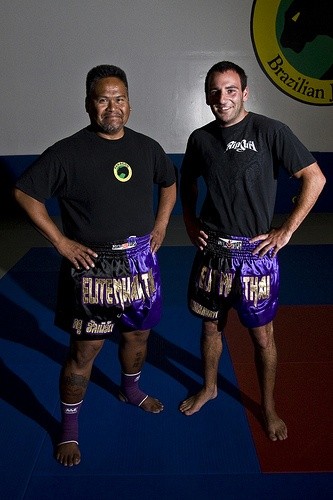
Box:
[178,61,326,444]
[14,64,178,468]
